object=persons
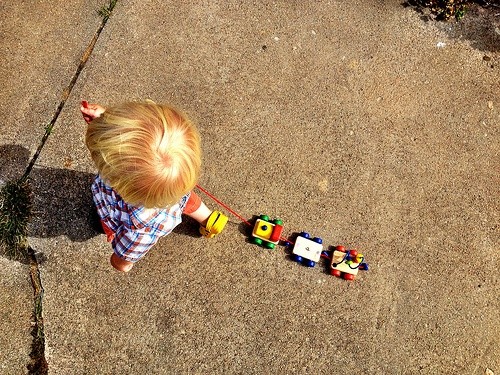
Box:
[75,98,228,272]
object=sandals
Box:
[199,210,228,239]
